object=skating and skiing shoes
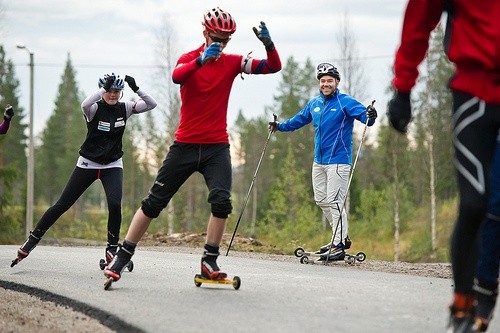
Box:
[470,275,498,333]
[194,246,241,290]
[103,250,130,290]
[10,230,42,268]
[99,241,133,272]
[446,299,479,333]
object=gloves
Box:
[103,76,116,93]
[124,75,139,93]
[387,90,411,134]
[197,42,222,67]
[268,121,279,134]
[252,21,275,52]
[3,104,14,122]
[364,105,377,119]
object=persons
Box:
[268,63,378,262]
[386,0,500,333]
[0,103,14,135]
[103,6,282,282]
[18,72,157,263]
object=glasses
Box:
[207,32,231,43]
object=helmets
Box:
[202,6,237,34]
[97,72,124,90]
[317,63,340,82]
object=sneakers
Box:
[320,237,351,252]
[319,244,345,261]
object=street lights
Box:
[15,46,33,240]
